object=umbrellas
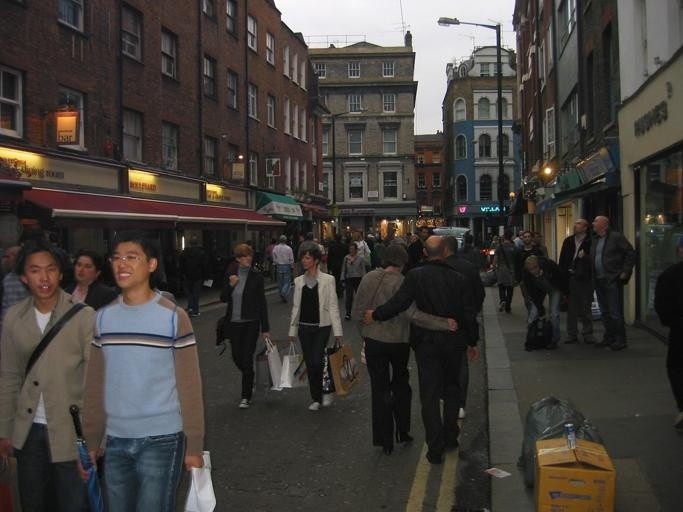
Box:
[68,405,104,512]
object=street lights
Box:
[255,337,310,392]
[321,337,367,395]
[215,316,232,357]
[183,450,218,512]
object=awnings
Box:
[255,192,303,221]
[26,188,286,225]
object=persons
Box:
[558,218,597,344]
[326,227,430,320]
[483,231,547,314]
[286,240,344,411]
[519,255,563,344]
[208,241,224,289]
[0,244,28,326]
[217,244,271,409]
[457,235,483,275]
[652,235,683,431]
[266,232,326,303]
[75,227,204,511]
[178,234,206,317]
[589,216,637,351]
[361,234,482,464]
[348,245,458,457]
[0,239,98,512]
[62,250,114,308]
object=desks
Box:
[328,104,368,214]
[434,13,514,243]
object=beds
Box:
[68,405,104,512]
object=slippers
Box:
[524,318,556,351]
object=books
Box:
[536,437,617,512]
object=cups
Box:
[109,255,146,266]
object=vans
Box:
[395,428,415,444]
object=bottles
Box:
[279,234,287,241]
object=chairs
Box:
[498,301,512,312]
[425,446,444,465]
[457,407,466,419]
[184,308,201,317]
[322,391,335,407]
[443,435,460,450]
[308,400,321,411]
[546,330,629,351]
[344,314,352,321]
[237,397,252,408]
[382,441,394,456]
[280,294,288,304]
